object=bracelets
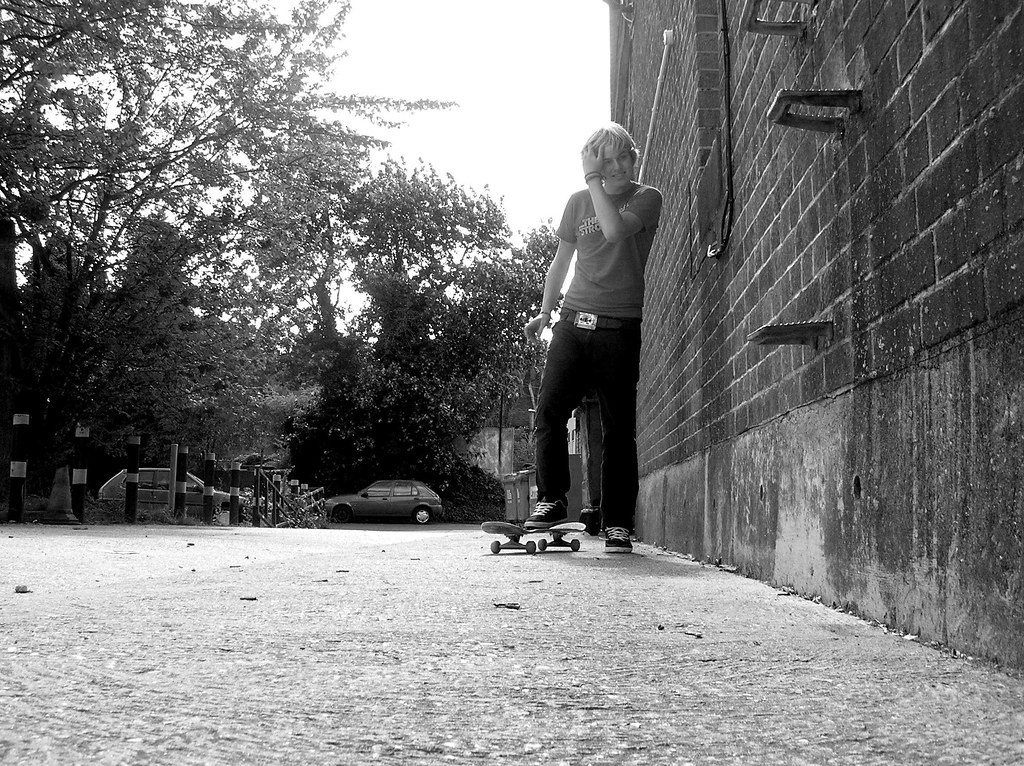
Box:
[584,171,603,184]
[541,312,551,323]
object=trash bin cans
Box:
[501,469,536,523]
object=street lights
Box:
[493,385,520,475]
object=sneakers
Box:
[523,498,569,527]
[603,525,633,554]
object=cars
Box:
[325,479,442,525]
[97,467,246,521]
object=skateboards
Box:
[480,521,586,554]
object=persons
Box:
[524,120,662,554]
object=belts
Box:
[561,311,643,329]
